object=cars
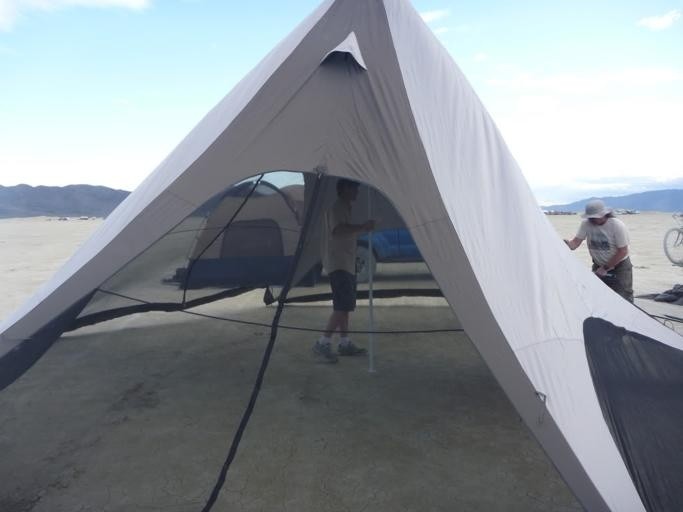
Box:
[356,229,423,285]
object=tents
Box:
[4,0,683,512]
[167,167,335,290]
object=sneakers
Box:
[337,341,368,356]
[313,341,337,364]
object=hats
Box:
[581,199,612,219]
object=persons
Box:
[559,196,636,306]
[307,178,370,364]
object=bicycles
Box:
[664,213,683,264]
